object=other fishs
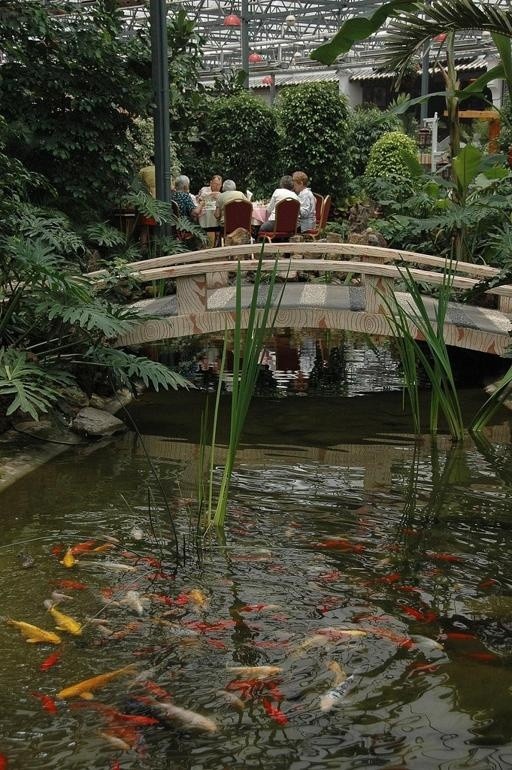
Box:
[0,501,511,770]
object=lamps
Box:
[223,0,242,27]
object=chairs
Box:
[170,191,333,260]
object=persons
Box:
[139,150,316,241]
